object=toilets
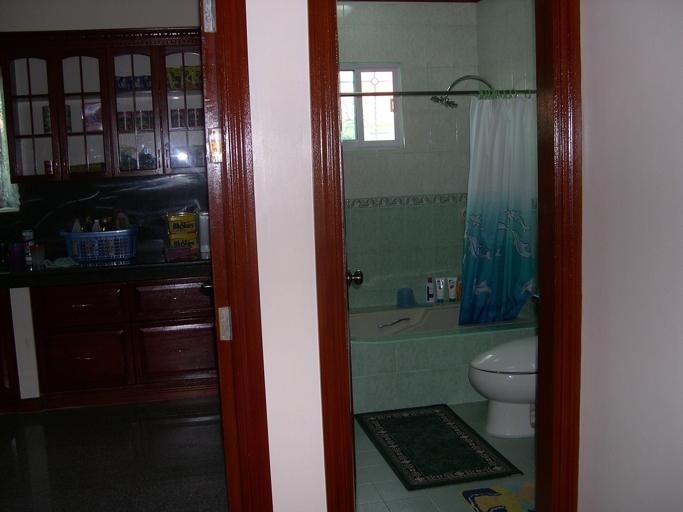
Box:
[468,294,538,437]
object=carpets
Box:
[354,401,523,491]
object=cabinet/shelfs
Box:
[1,29,112,184]
[129,277,219,406]
[112,25,207,179]
[0,280,136,415]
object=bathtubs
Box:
[349,306,536,416]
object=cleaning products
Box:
[71,211,129,255]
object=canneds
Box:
[170,108,203,127]
[44,160,53,174]
[116,111,153,129]
[22,229,35,268]
[165,211,211,260]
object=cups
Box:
[398,287,415,307]
[31,243,46,272]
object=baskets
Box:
[58,221,143,265]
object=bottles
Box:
[9,229,34,269]
[72,212,128,261]
[199,211,210,261]
[117,73,205,170]
[425,276,435,305]
[43,103,72,175]
[456,278,462,303]
